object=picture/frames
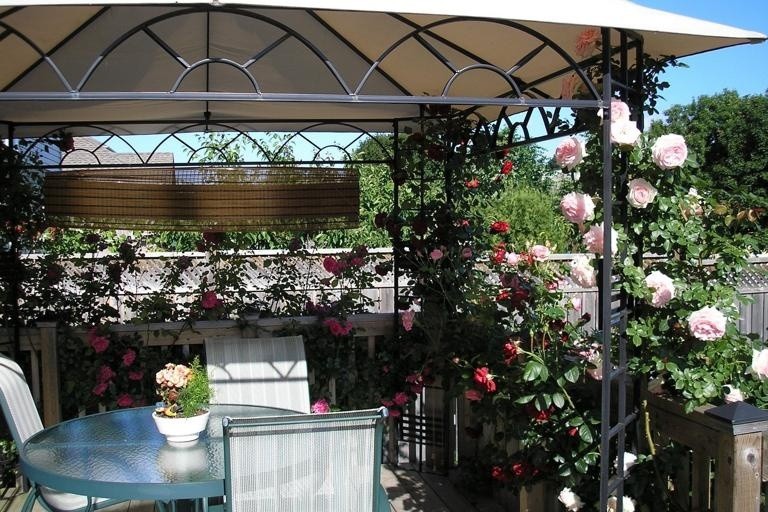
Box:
[18,404,306,512]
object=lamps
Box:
[222,405,391,512]
[203,334,310,415]
[0,351,127,512]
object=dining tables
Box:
[152,354,216,443]
